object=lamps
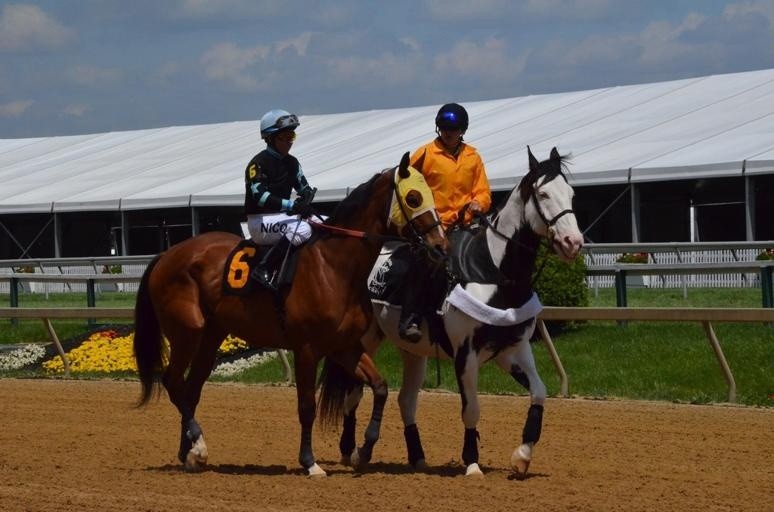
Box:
[110,231,115,255]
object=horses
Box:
[312,143,586,482]
[127,146,452,482]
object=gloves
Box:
[292,198,306,214]
[300,187,314,199]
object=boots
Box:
[250,235,298,292]
[398,263,428,345]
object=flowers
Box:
[614,251,648,265]
[753,247,772,260]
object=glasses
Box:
[278,132,297,143]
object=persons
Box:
[398,103,492,343]
[244,109,330,290]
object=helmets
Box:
[435,103,469,132]
[259,108,300,140]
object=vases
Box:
[756,271,772,283]
[626,275,648,286]
